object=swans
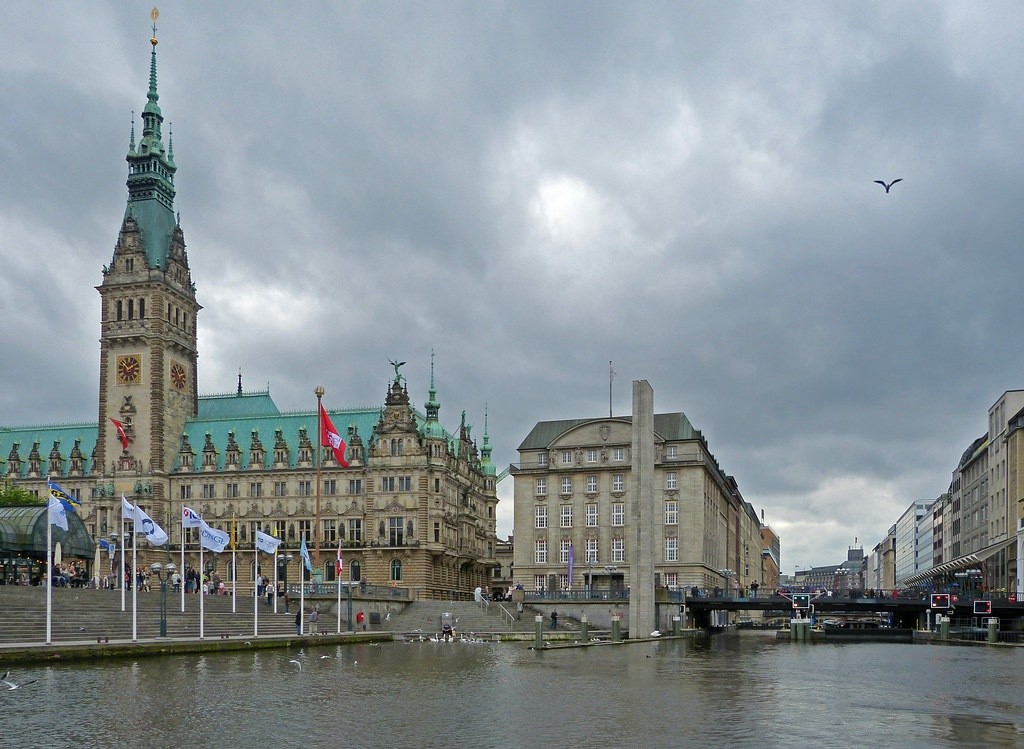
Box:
[429,632,490,643]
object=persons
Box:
[309,574,397,596]
[35,561,280,607]
[443,621,452,637]
[563,581,746,599]
[777,584,1017,603]
[285,593,367,635]
[484,580,558,629]
[749,580,759,599]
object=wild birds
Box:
[873,178,904,194]
[289,659,302,671]
[385,612,392,622]
[0,671,38,691]
[651,631,662,637]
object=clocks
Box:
[168,358,190,395]
[115,353,142,386]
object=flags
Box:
[48,480,81,513]
[123,497,134,521]
[48,494,69,531]
[231,518,235,551]
[256,525,282,554]
[135,504,169,546]
[201,519,229,553]
[337,541,343,576]
[320,403,349,469]
[182,506,201,527]
[300,533,313,572]
[109,418,128,449]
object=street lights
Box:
[110,530,131,590]
[150,562,178,638]
[277,553,294,613]
